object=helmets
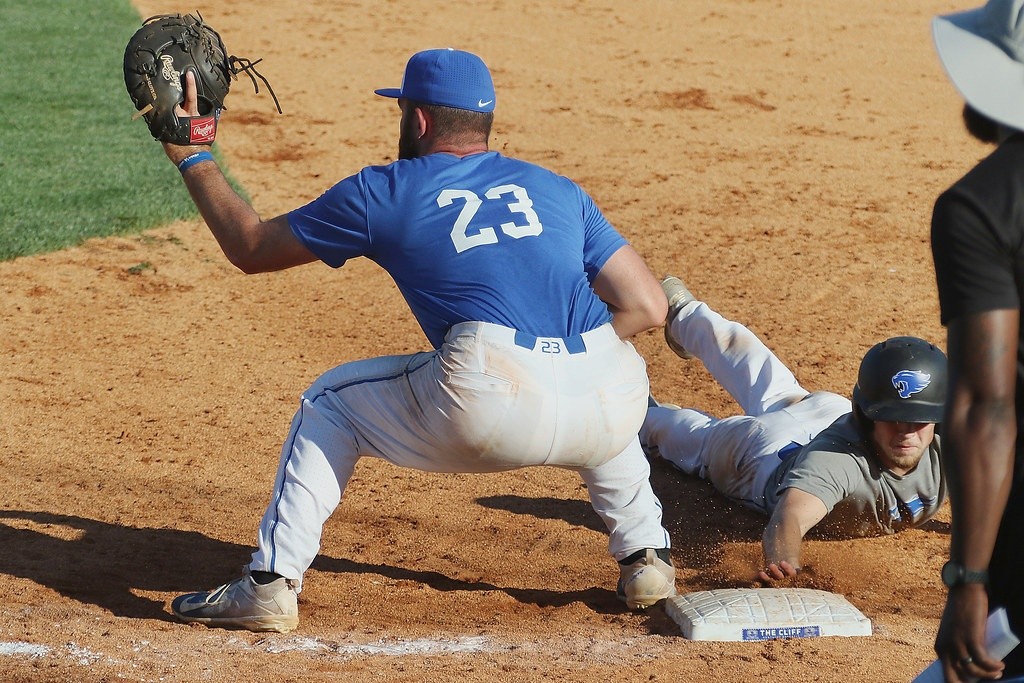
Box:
[856,337,949,423]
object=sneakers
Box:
[616,548,677,610]
[171,574,300,634]
[660,275,700,359]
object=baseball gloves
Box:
[120,8,281,146]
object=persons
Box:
[642,278,948,587]
[162,49,677,633]
[930,0,1024,683]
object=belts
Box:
[515,331,586,354]
[778,441,802,463]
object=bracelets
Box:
[178,153,212,173]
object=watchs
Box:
[941,561,989,586]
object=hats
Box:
[374,48,495,113]
[932,0,1024,131]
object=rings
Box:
[961,655,972,665]
